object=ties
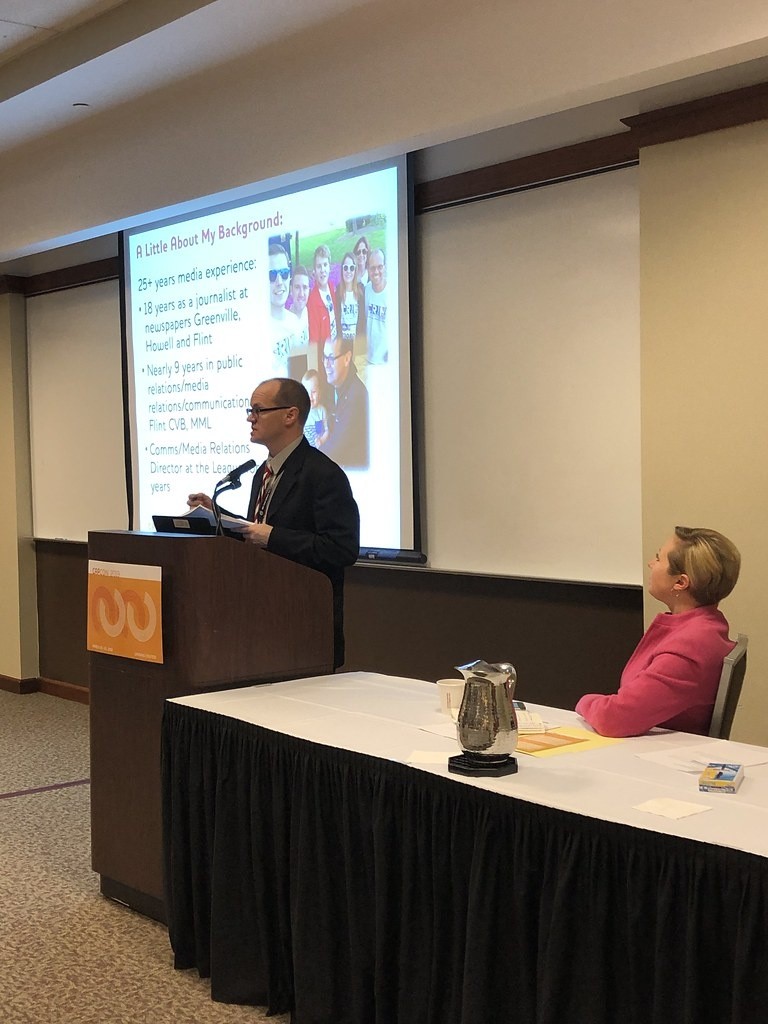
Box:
[253,467,272,524]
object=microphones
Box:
[217,460,256,486]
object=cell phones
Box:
[512,702,526,711]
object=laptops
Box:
[152,515,214,536]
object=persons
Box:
[574,525,741,737]
[186,378,360,670]
[270,235,393,469]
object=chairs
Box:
[710,632,750,741]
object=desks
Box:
[160,670,768,1024]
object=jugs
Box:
[455,659,516,764]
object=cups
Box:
[436,679,464,713]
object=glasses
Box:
[343,264,356,271]
[269,268,290,282]
[246,406,291,418]
[322,354,344,364]
[357,248,368,255]
